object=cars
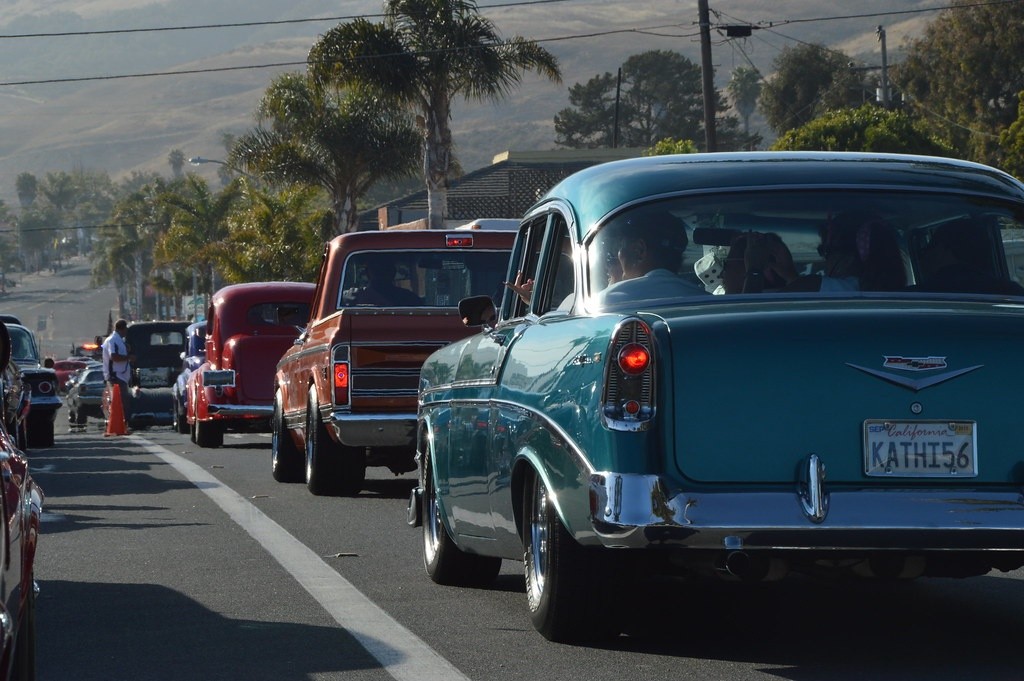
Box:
[46,343,107,429]
[171,320,212,433]
[0,318,45,681]
[0,313,22,326]
[101,320,195,429]
[184,281,320,448]
[267,228,577,499]
[407,148,1024,641]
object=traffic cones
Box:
[105,384,130,435]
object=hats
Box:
[605,208,688,253]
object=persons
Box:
[103,319,136,432]
[503,204,907,310]
[349,259,423,305]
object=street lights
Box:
[188,156,260,193]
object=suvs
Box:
[0,324,63,447]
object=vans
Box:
[426,218,540,309]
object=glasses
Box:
[480,314,495,324]
[817,244,836,259]
